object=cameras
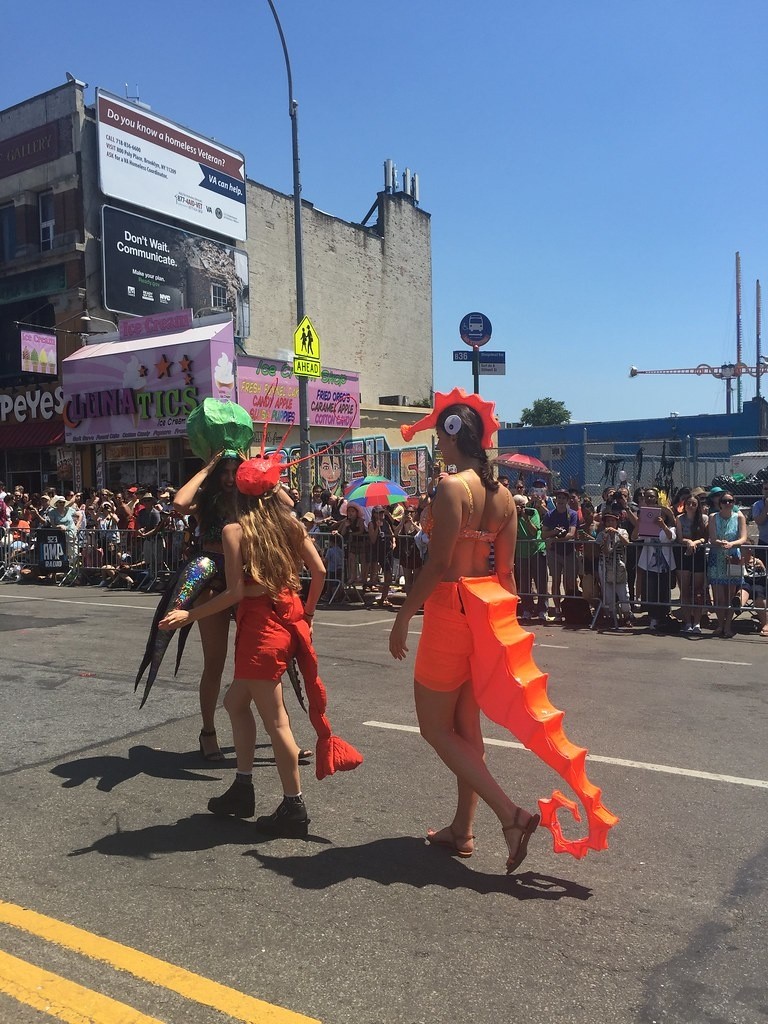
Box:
[104,504,109,508]
[557,529,567,538]
[612,491,622,499]
[520,505,535,516]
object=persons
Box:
[172,450,316,763]
[388,389,541,877]
[0,480,767,635]
[157,456,328,838]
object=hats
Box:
[321,491,385,517]
[300,512,315,521]
[603,505,677,526]
[40,496,71,507]
[554,488,569,496]
[691,487,725,496]
[101,501,116,512]
[533,479,547,486]
[128,487,170,504]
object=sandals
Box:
[427,827,475,858]
[502,807,540,874]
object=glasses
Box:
[302,518,309,523]
[720,499,734,504]
[687,502,696,507]
[515,486,523,490]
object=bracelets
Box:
[303,611,314,617]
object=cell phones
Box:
[577,529,584,534]
[404,510,409,515]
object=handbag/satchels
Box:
[97,536,107,550]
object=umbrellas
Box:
[489,453,550,496]
[347,481,407,523]
[343,475,391,499]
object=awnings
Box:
[0,422,65,448]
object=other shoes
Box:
[130,577,172,591]
[594,612,768,641]
[732,597,742,615]
[363,585,394,608]
[521,611,562,622]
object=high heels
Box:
[199,729,225,762]
[298,750,314,759]
[257,802,311,838]
[208,780,255,818]
